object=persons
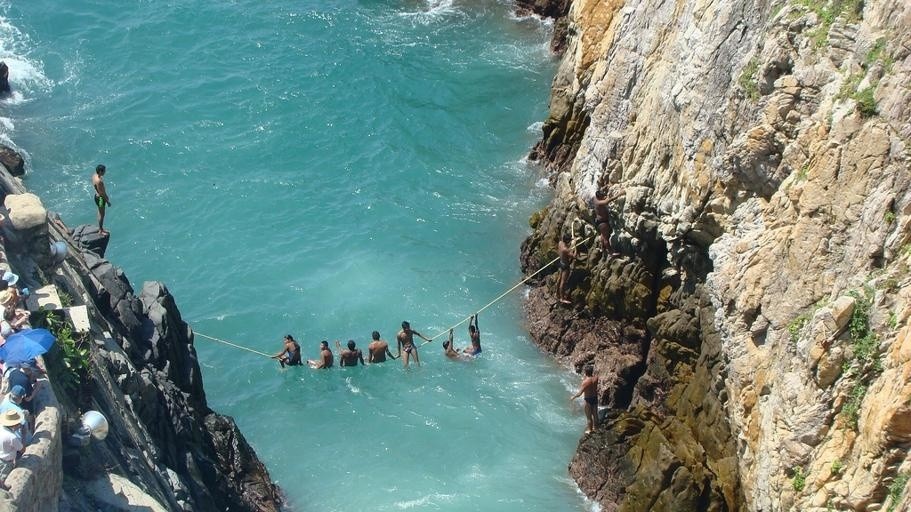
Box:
[336,338,365,367]
[463,313,483,356]
[307,340,334,371]
[365,331,397,364]
[570,364,602,433]
[92,163,112,237]
[557,232,578,305]
[443,329,473,362]
[271,334,304,369]
[592,185,627,259]
[0,252,33,492]
[396,320,433,368]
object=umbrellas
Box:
[1,326,57,366]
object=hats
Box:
[0,290,11,304]
[2,272,18,287]
[11,385,25,396]
[0,410,22,427]
[19,361,39,370]
